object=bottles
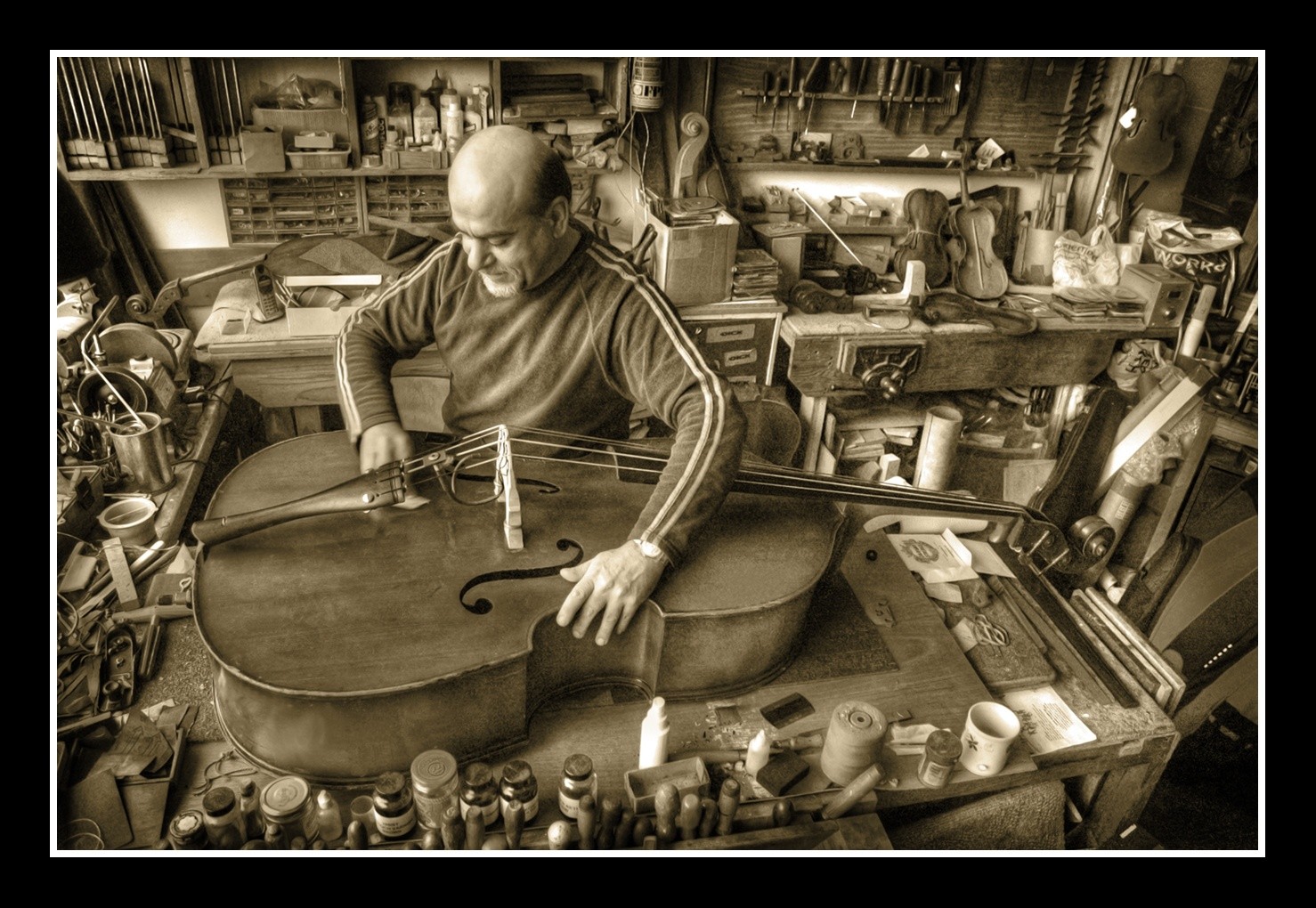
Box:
[168,697,770,850]
[359,69,483,155]
[916,730,962,789]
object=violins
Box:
[951,139,1008,301]
[893,189,950,289]
[1205,60,1257,180]
[1110,56,1188,177]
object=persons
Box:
[331,124,749,647]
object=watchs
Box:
[633,539,670,567]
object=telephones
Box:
[252,266,284,322]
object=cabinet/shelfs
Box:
[58,58,634,180]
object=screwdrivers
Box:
[907,68,919,128]
[772,76,782,128]
[787,57,796,132]
[763,73,769,103]
[851,57,871,120]
[884,57,904,128]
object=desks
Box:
[207,274,371,438]
[91,520,1181,851]
[770,288,1237,470]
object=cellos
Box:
[190,428,1118,785]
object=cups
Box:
[957,701,1021,776]
[106,411,188,495]
